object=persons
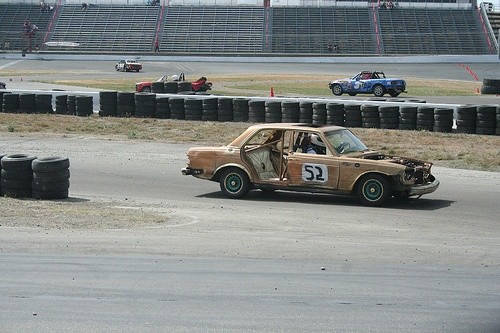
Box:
[146,0,160,7]
[375,0,400,10]
[24,19,30,30]
[306,133,338,156]
[82,3,87,10]
[370,73,377,79]
[154,39,160,52]
[40,0,47,10]
[4,41,10,49]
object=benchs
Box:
[301,137,310,153]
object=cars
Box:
[329,71,406,96]
[182,123,440,206]
[136,73,212,93]
[115,59,142,72]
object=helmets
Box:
[310,133,326,147]
[172,75,179,81]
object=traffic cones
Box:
[476,88,480,93]
[269,87,274,97]
